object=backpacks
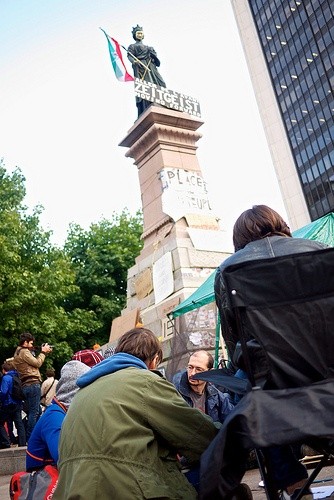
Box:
[3,373,22,402]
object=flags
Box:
[100,28,135,83]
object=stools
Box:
[264,433,334,499]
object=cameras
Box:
[42,343,55,349]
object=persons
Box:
[14,333,51,443]
[126,24,166,115]
[0,361,29,447]
[0,424,19,448]
[170,346,240,490]
[25,359,92,476]
[51,326,223,499]
[39,365,60,411]
[214,205,334,500]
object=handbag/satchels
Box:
[9,465,59,500]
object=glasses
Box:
[185,366,207,373]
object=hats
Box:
[73,349,105,368]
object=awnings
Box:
[170,212,334,371]
